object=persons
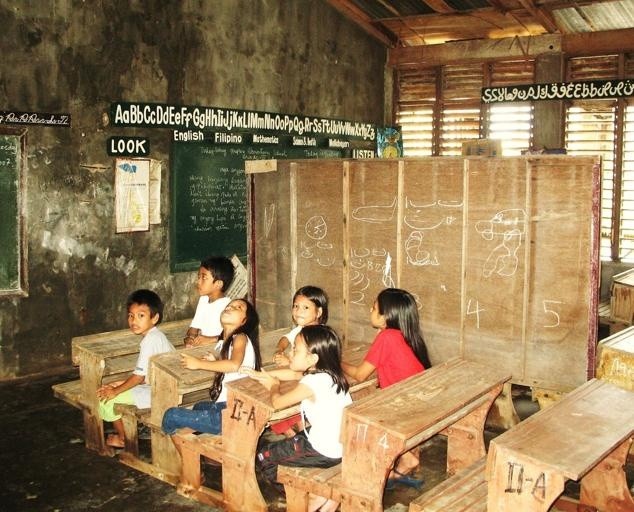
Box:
[239,323,354,511]
[339,288,430,483]
[159,297,261,485]
[182,255,232,348]
[93,288,174,448]
[270,283,328,366]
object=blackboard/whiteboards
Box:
[171,128,349,273]
[0,124,28,299]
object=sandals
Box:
[385,464,424,488]
